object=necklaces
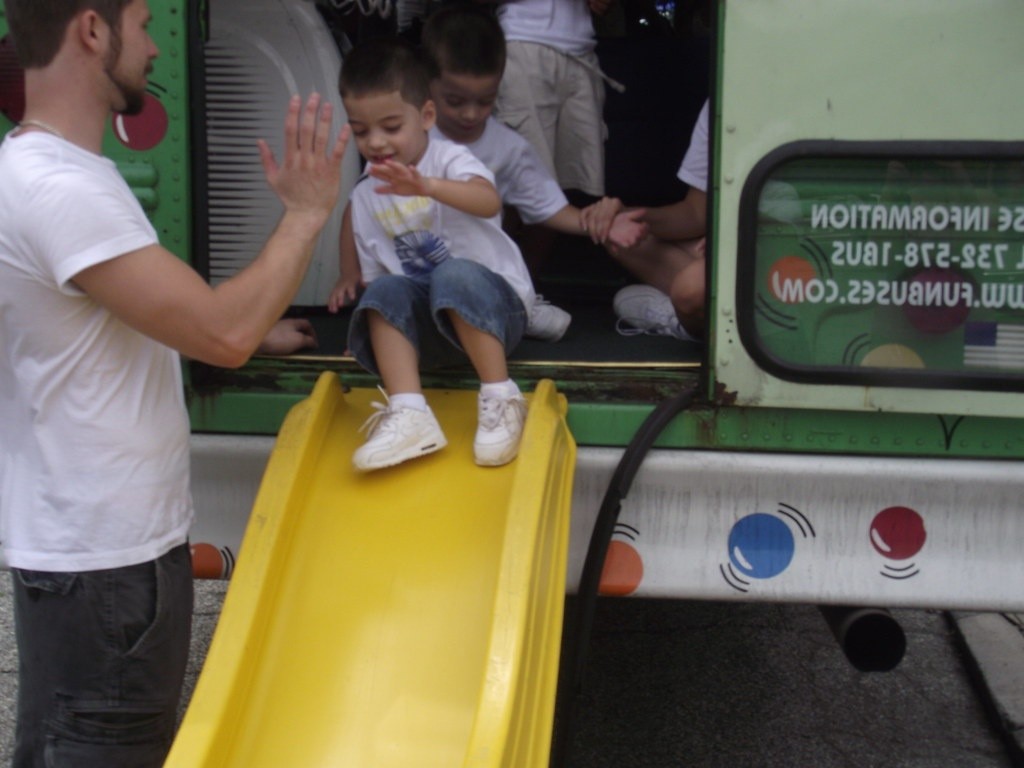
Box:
[17,115,68,144]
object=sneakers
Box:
[613,284,690,340]
[525,300,572,341]
[351,405,448,473]
[472,395,529,467]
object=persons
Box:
[325,5,650,343]
[0,0,353,768]
[575,97,710,345]
[485,0,627,296]
[325,35,535,475]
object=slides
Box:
[157,369,577,768]
